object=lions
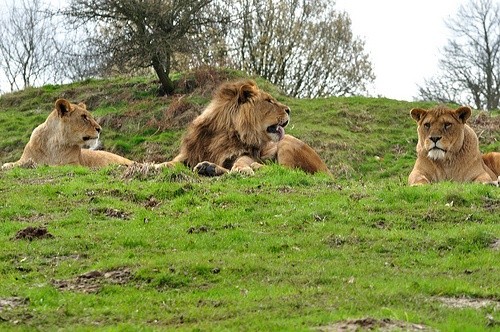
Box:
[1,96,151,171]
[147,79,337,179]
[407,105,500,186]
